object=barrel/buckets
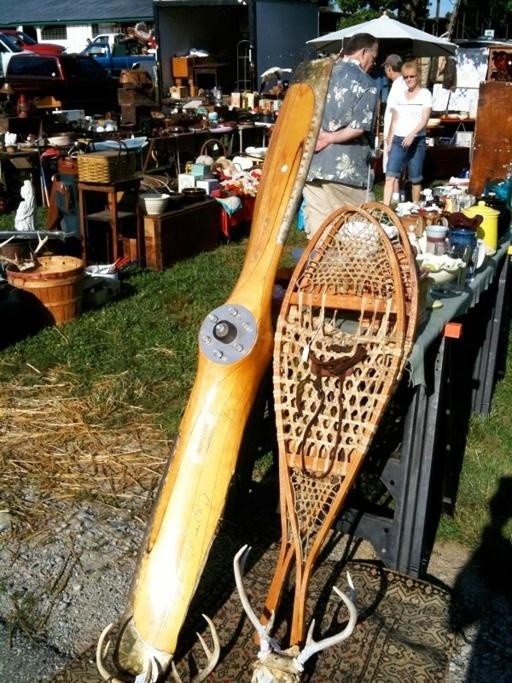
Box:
[5,255,86,324]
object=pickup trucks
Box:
[0,30,157,114]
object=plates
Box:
[335,219,398,244]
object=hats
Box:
[381,54,403,67]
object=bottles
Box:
[192,84,287,126]
[424,136,430,146]
[15,93,120,149]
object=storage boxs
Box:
[77,150,143,184]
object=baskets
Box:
[77,137,136,183]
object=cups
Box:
[389,177,502,298]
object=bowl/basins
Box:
[139,192,170,214]
[182,187,206,199]
[427,117,441,127]
[29,43,66,56]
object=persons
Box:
[302,32,379,249]
[380,54,404,204]
[382,60,433,215]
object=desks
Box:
[441,116,474,144]
[173,53,228,96]
[144,119,271,174]
[273,176,512,585]
[77,181,144,269]
[142,193,255,271]
[0,144,85,208]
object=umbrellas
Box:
[305,10,459,156]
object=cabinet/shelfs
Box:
[236,40,253,91]
